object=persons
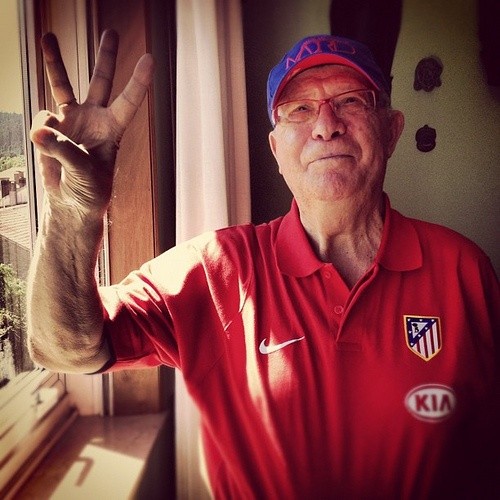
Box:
[23,22,500,500]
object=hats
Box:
[267,34,391,129]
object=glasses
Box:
[270,86,387,128]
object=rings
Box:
[57,97,76,110]
